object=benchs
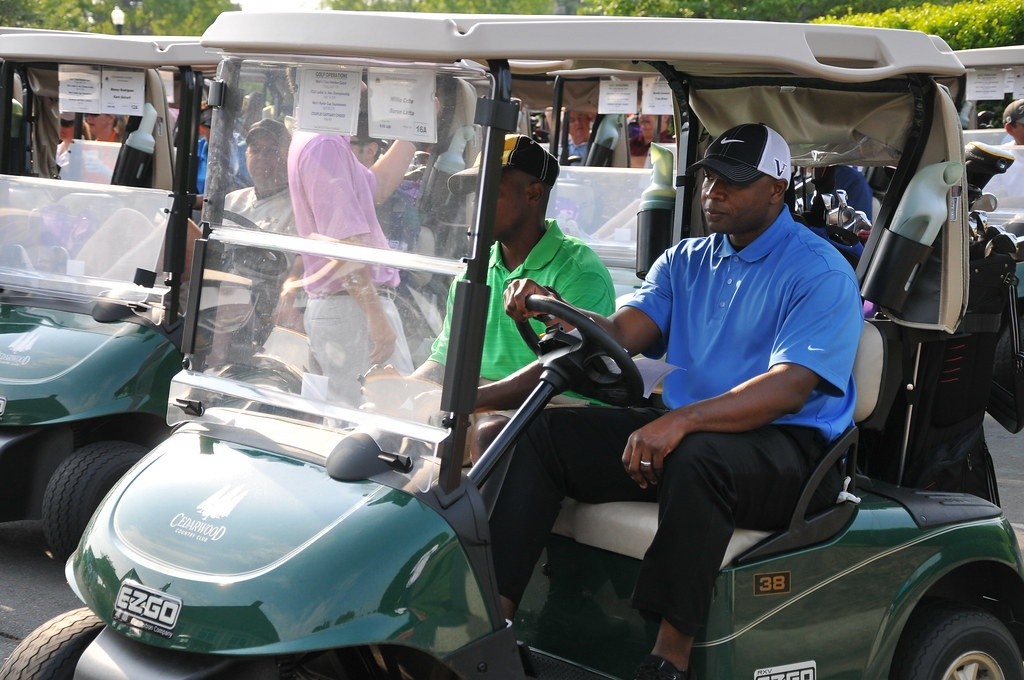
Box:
[545,166,653,297]
[462,321,906,571]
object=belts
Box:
[333,291,396,300]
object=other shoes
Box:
[634,653,686,680]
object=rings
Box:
[640,460,651,466]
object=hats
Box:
[685,121,791,191]
[59,112,75,121]
[1003,98,1024,124]
[245,118,291,147]
[448,135,560,195]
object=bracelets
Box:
[533,285,563,321]
[411,140,422,147]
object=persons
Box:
[792,162,873,256]
[488,124,861,680]
[357,131,617,465]
[982,99,1024,207]
[559,103,676,170]
[284,66,442,426]
[52,99,303,379]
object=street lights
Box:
[110,5,128,35]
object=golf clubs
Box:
[795,178,872,234]
[968,183,1024,260]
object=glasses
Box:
[60,119,73,127]
